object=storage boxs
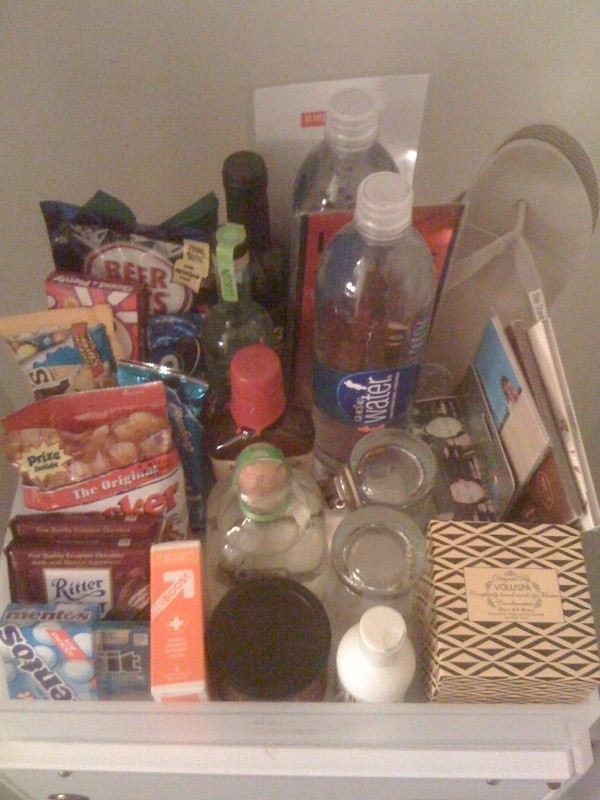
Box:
[150,539,215,702]
[48,271,149,361]
[97,621,148,690]
[0,601,99,701]
[412,519,600,704]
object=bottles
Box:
[193,82,437,705]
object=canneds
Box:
[206,573,333,702]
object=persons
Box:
[499,375,522,412]
